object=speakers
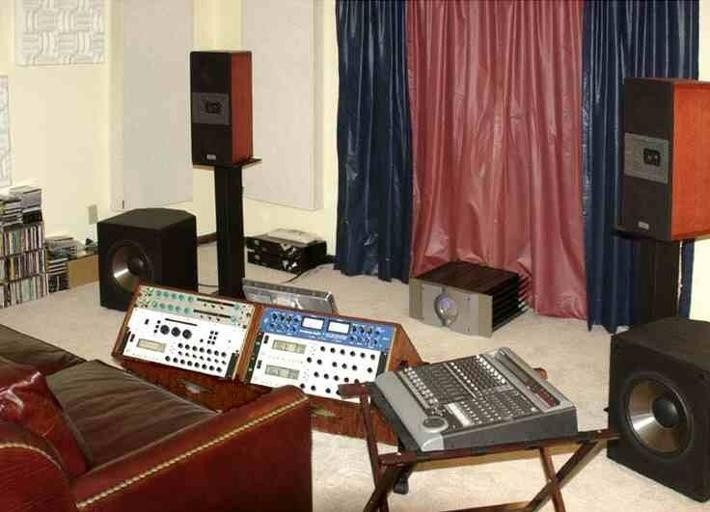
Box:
[96,206,199,312]
[189,50,254,166]
[620,76,710,243]
[604,314,710,503]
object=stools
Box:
[338,366,626,512]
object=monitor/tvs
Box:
[240,277,339,315]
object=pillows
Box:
[0,355,94,478]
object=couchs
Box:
[0,321,319,512]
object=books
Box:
[0,185,79,310]
